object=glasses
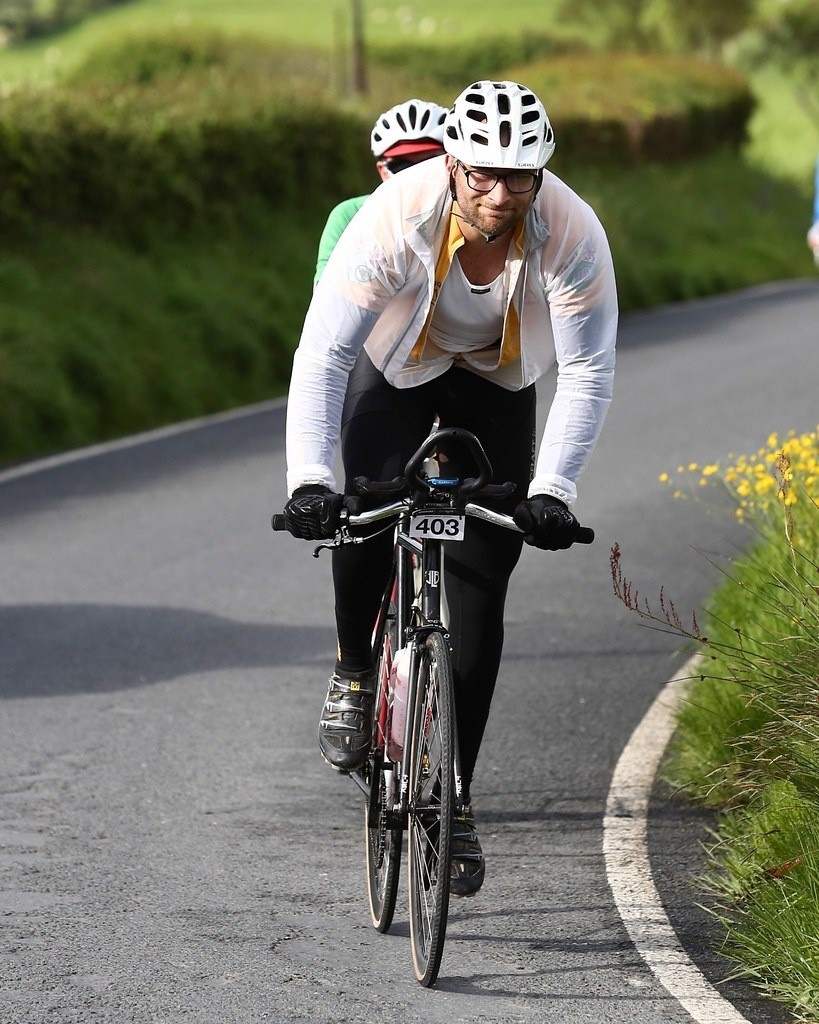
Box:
[382,159,414,175]
[458,159,539,193]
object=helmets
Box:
[370,99,450,161]
[443,81,556,169]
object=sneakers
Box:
[434,797,485,895]
[319,665,375,772]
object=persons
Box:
[806,157,818,261]
[315,100,447,293]
[284,69,620,905]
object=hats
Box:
[383,137,442,157]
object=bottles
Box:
[390,634,418,761]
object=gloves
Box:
[282,483,365,541]
[513,494,580,551]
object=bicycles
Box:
[269,407,596,989]
